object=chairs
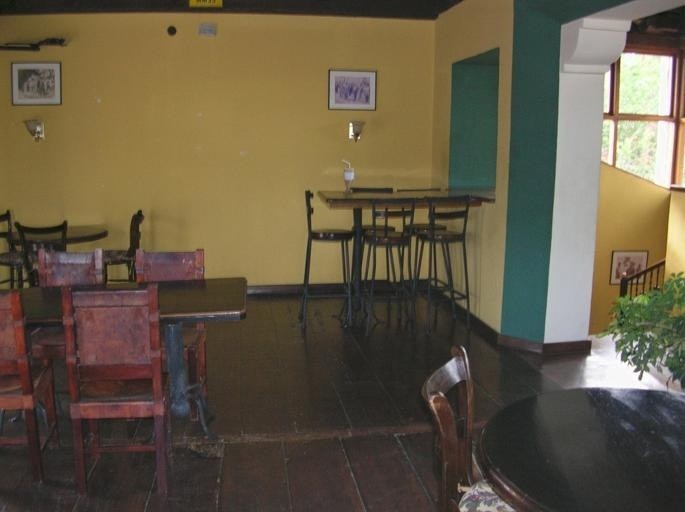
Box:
[1,288,60,485]
[0,210,205,423]
[62,282,173,496]
[296,190,470,337]
[421,344,515,511]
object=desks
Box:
[475,388,684,512]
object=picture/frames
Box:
[328,69,376,110]
[609,250,648,285]
[11,61,61,106]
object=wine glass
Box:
[343,167,355,196]
